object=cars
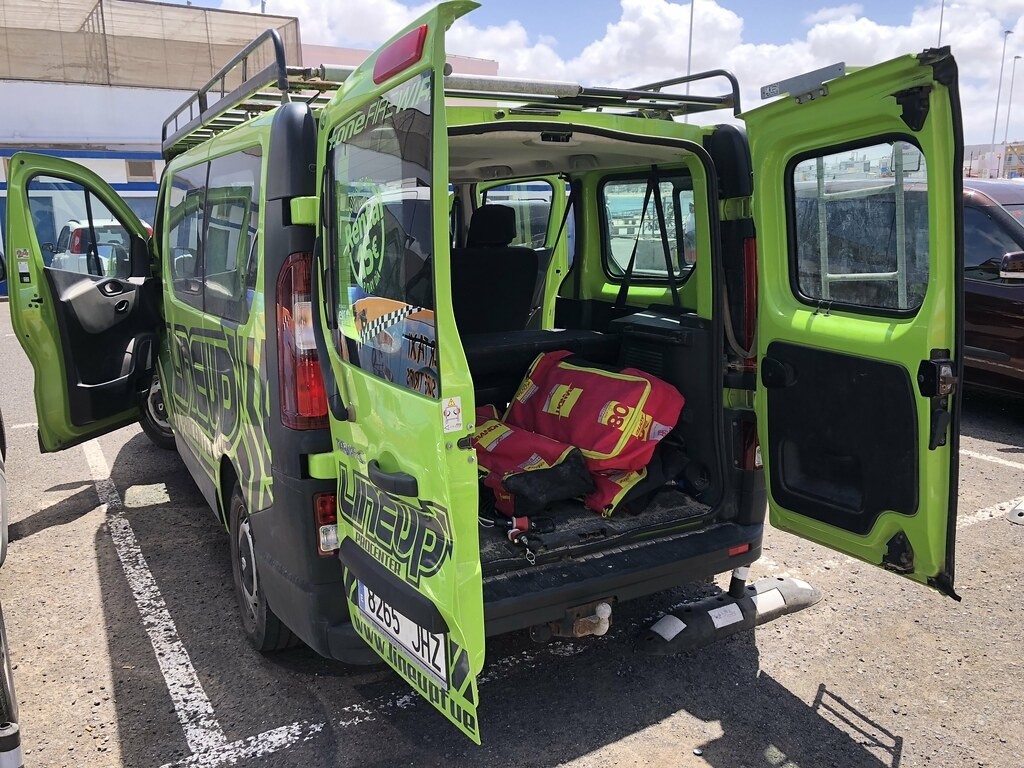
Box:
[672,178,1024,406]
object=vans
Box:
[0,1,968,749]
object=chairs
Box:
[451,206,538,334]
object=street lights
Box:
[990,31,1014,159]
[664,1,695,124]
[1002,56,1022,142]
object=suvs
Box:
[38,214,154,276]
